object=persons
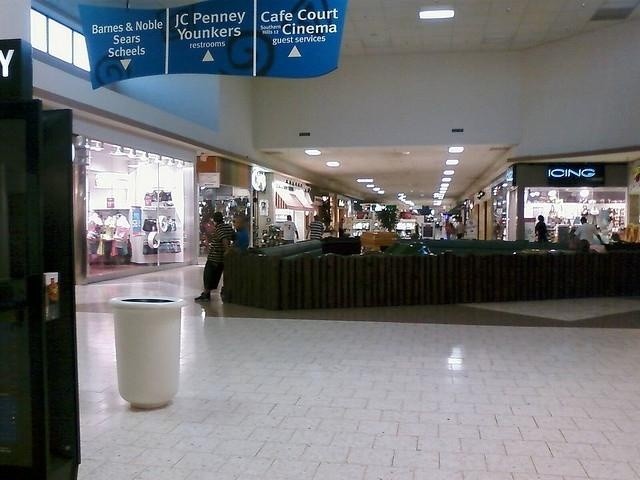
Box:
[444,218,465,241]
[571,216,603,248]
[232,215,251,249]
[533,214,551,244]
[339,217,345,237]
[305,215,324,241]
[277,214,299,245]
[192,212,236,304]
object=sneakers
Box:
[194,292,210,302]
